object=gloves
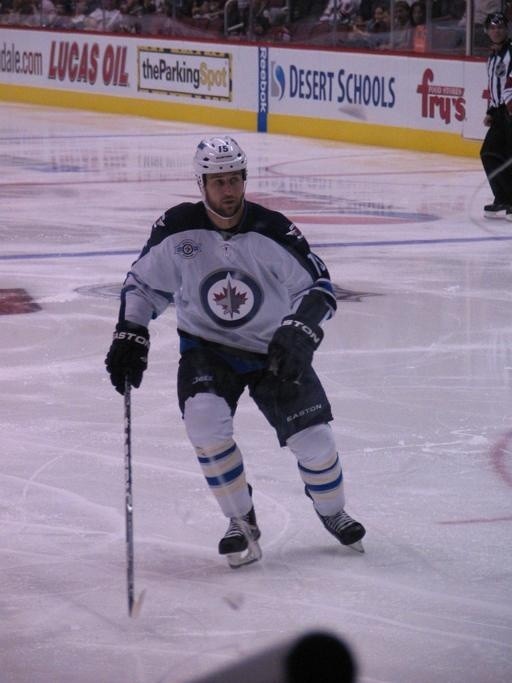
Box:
[105,320,149,395]
[269,315,323,379]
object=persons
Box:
[479,12,512,215]
[105,136,366,556]
[1,1,512,51]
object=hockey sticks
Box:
[125,377,147,618]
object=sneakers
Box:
[320,509,365,545]
[219,509,259,554]
[484,203,512,214]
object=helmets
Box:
[484,12,507,27]
[194,136,246,220]
